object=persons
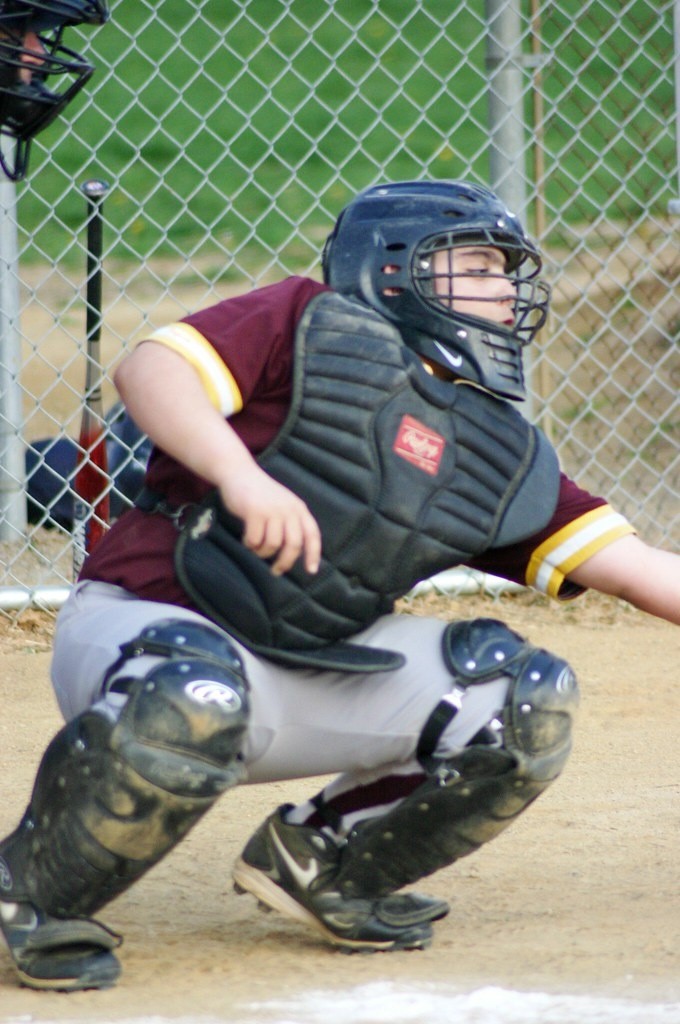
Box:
[0,0,109,183]
[1,182,680,992]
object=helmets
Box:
[0,0,112,185]
[320,179,554,402]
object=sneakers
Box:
[230,802,435,955]
[1,898,126,993]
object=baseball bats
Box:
[63,174,118,600]
